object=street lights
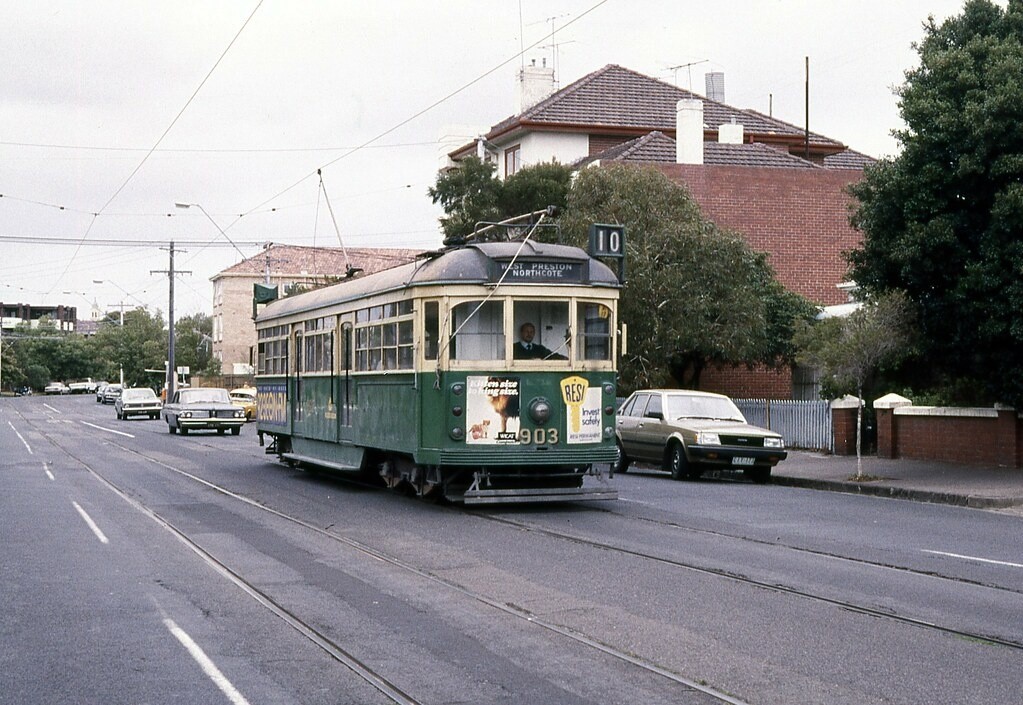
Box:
[62,290,124,383]
[176,201,274,284]
[93,277,148,312]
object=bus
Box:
[248,204,628,504]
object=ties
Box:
[527,344,531,350]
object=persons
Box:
[513,323,569,360]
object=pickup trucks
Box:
[69,377,97,394]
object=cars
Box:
[115,387,163,420]
[45,382,70,395]
[614,389,788,484]
[162,388,247,435]
[230,388,257,422]
[97,381,125,404]
